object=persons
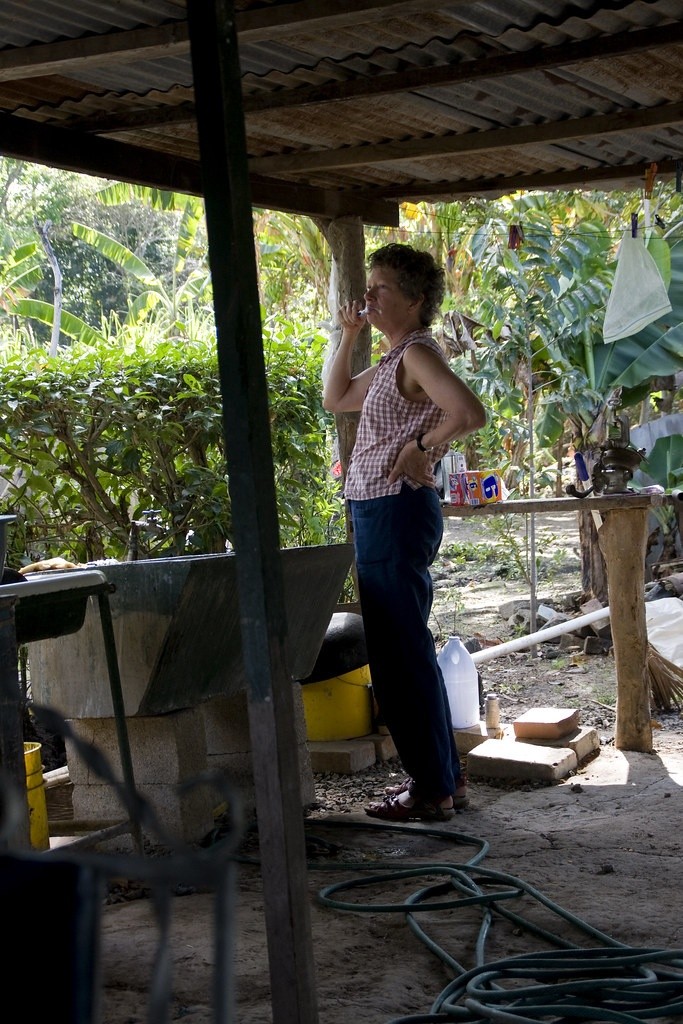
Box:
[322,242,489,821]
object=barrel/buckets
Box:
[301,665,372,741]
[437,636,480,729]
[24,742,51,852]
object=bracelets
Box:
[417,433,433,453]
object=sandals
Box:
[383,770,470,809]
[364,780,455,822]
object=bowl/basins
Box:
[301,612,369,684]
